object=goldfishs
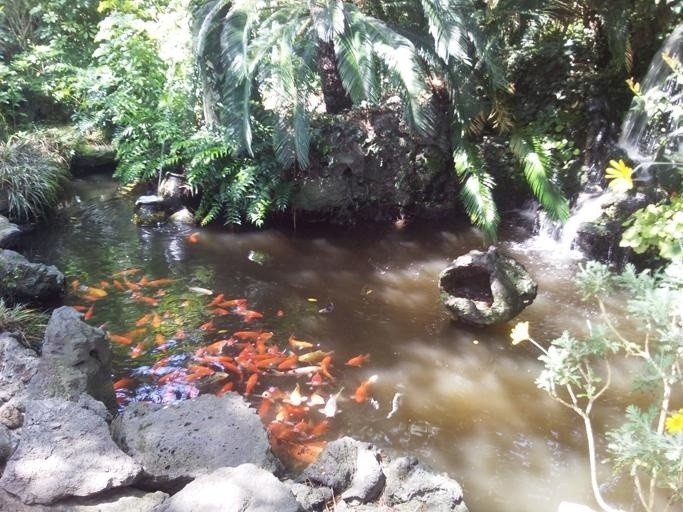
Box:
[68,267,379,467]
[188,232,200,244]
